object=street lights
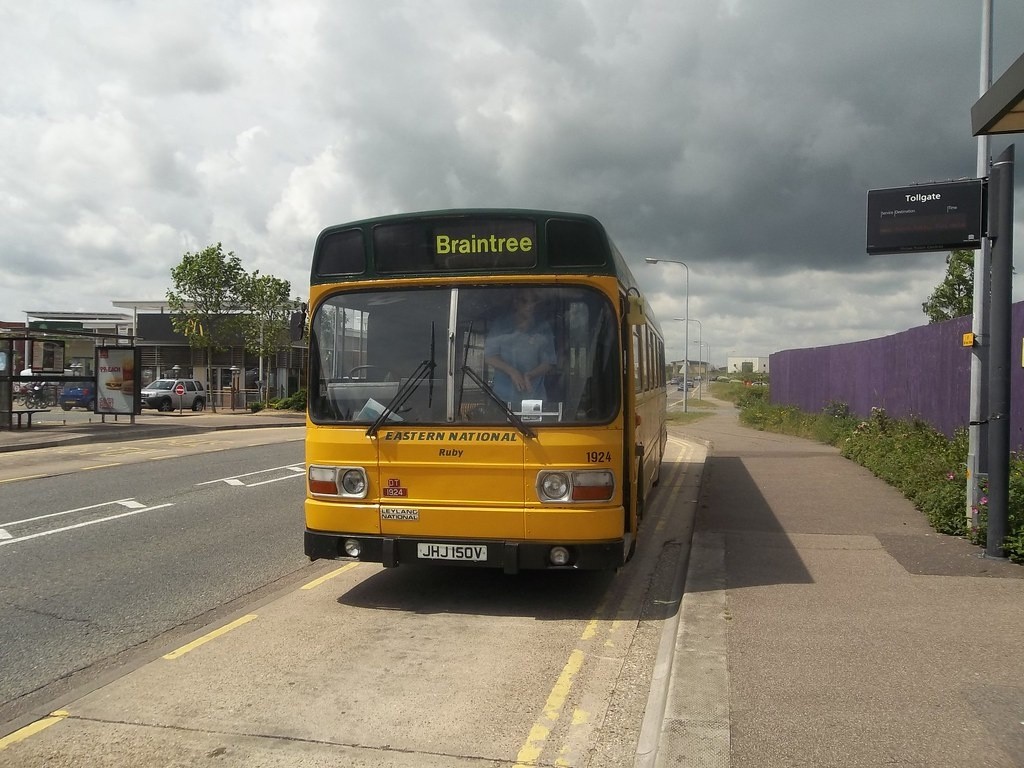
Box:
[673,317,702,403]
[172,365,181,378]
[693,339,710,392]
[645,257,688,413]
[229,364,240,410]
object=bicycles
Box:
[17,381,58,410]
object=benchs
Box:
[0,409,51,430]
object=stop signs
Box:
[176,385,184,395]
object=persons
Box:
[484,287,557,414]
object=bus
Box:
[297,206,668,579]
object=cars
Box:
[670,374,770,395]
[140,378,207,412]
[60,381,95,411]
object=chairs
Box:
[383,336,430,381]
